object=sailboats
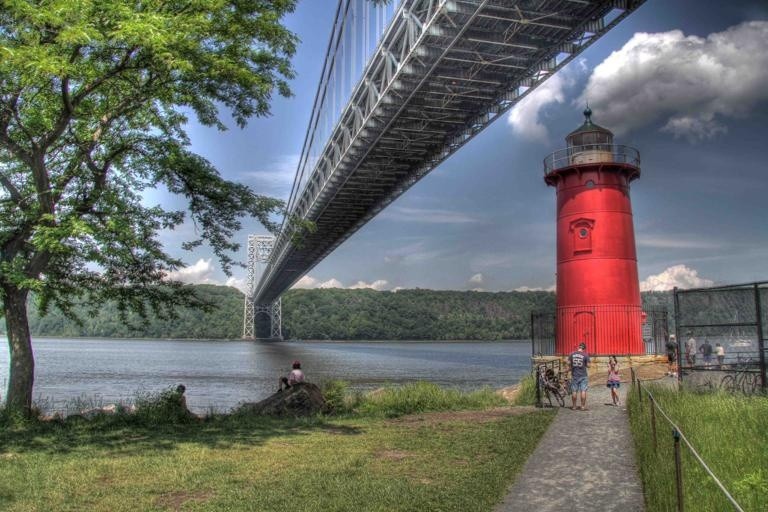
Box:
[725,308,754,349]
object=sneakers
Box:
[616,400,622,406]
[285,384,291,390]
[612,402,617,406]
[581,405,590,411]
[277,388,283,393]
[572,405,580,410]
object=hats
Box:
[292,360,301,365]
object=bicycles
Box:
[717,357,768,398]
[531,363,566,410]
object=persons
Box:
[606,354,622,406]
[276,360,305,392]
[714,343,725,366]
[567,342,592,410]
[175,384,187,407]
[698,337,712,370]
[665,334,677,378]
[684,330,697,367]
